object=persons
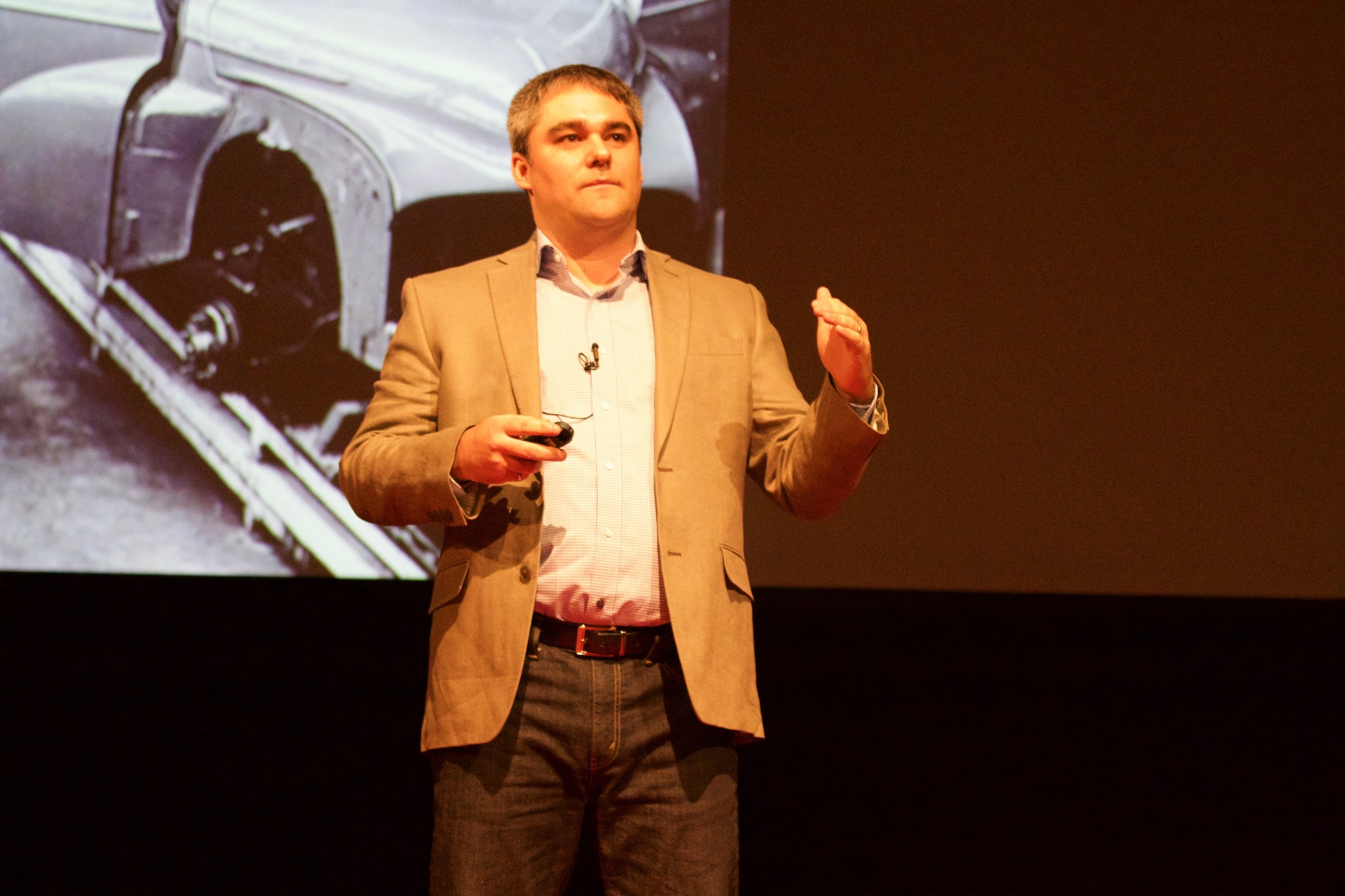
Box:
[338,67,891,894]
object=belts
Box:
[530,612,674,660]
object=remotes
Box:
[521,420,575,450]
